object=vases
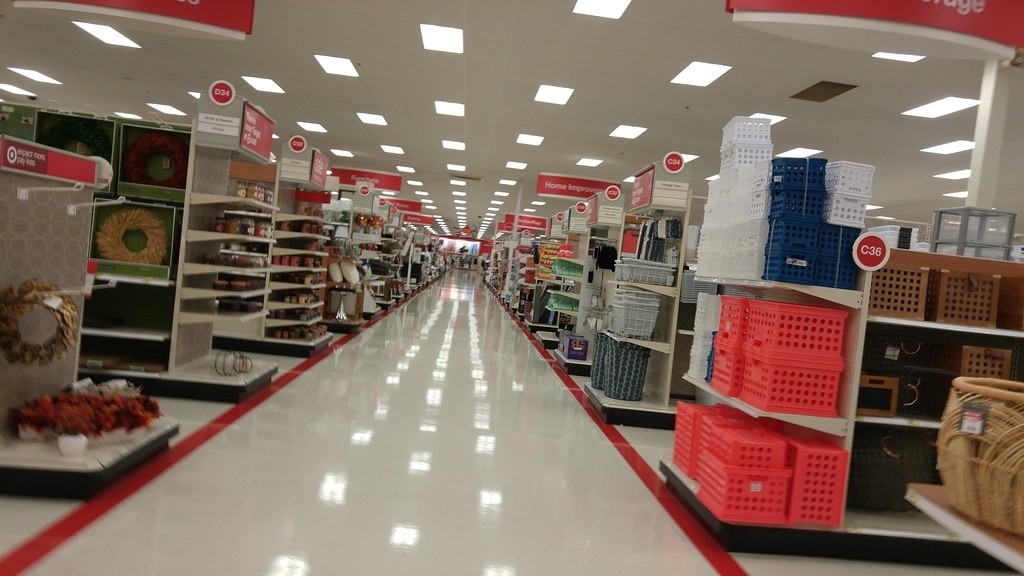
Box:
[20,419,167,456]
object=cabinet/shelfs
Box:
[657,239,1022,541]
[525,230,618,378]
[0,102,439,402]
[584,194,719,430]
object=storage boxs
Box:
[676,115,1016,531]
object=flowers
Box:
[12,386,164,435]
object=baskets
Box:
[934,376,1023,540]
